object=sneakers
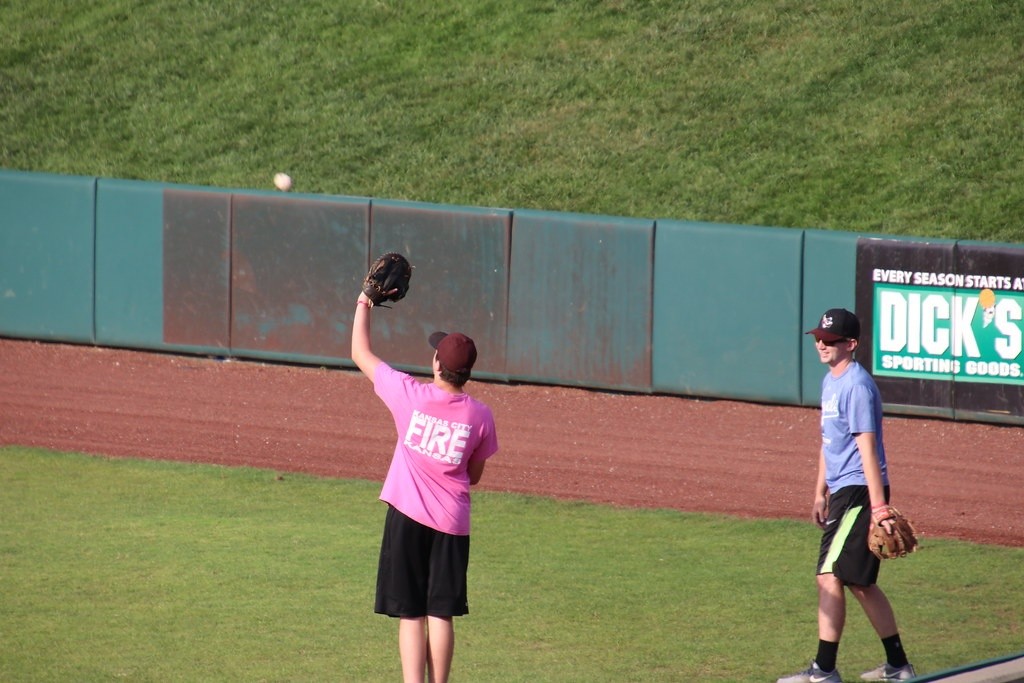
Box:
[860,663,915,682]
[777,660,843,683]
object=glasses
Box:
[815,335,848,346]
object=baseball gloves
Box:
[866,504,920,560]
[360,247,414,311]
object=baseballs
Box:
[273,172,293,192]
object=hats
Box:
[429,331,477,373]
[805,309,860,342]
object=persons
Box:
[351,253,498,683]
[777,309,916,683]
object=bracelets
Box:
[356,301,368,306]
